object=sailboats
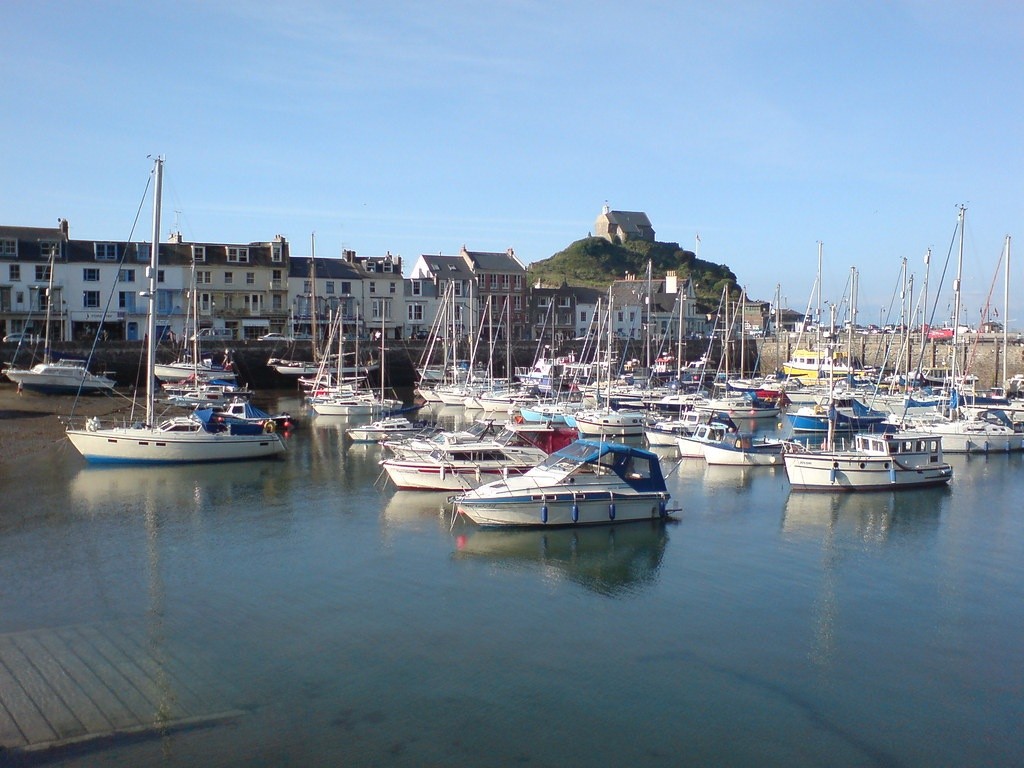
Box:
[412,279,676,435]
[785,302,955,490]
[675,206,1024,453]
[154,243,254,410]
[2,243,116,395]
[266,232,404,416]
[61,154,284,465]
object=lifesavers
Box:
[764,397,773,402]
[814,404,824,414]
[85,420,98,433]
[263,422,276,433]
[178,374,195,385]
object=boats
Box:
[345,417,420,441]
[216,397,292,427]
[383,419,551,491]
[644,408,788,467]
[448,439,682,532]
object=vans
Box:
[189,328,233,341]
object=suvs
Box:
[343,333,366,341]
[3,333,51,343]
[612,331,635,340]
[417,330,434,340]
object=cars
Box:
[257,333,295,341]
[575,334,594,341]
